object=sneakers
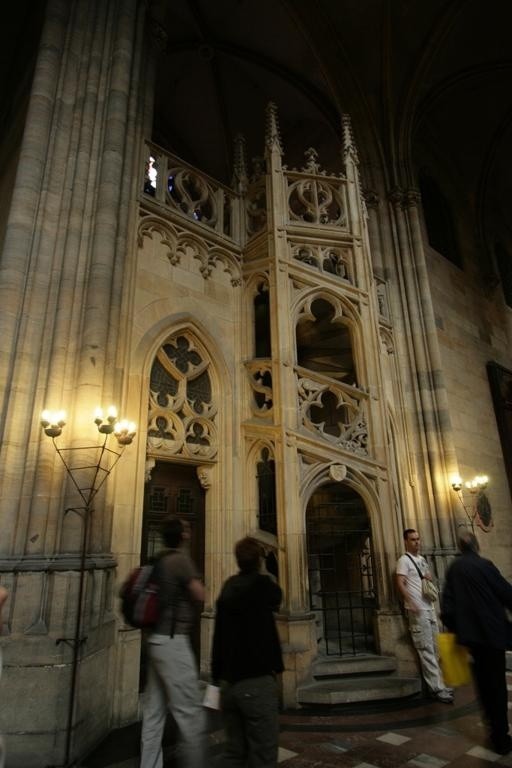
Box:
[429,688,455,703]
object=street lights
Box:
[449,473,490,554]
[38,404,139,766]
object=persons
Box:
[394,528,455,705]
[209,534,285,768]
[442,530,511,756]
[138,516,210,768]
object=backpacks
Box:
[119,549,182,628]
[422,577,439,601]
